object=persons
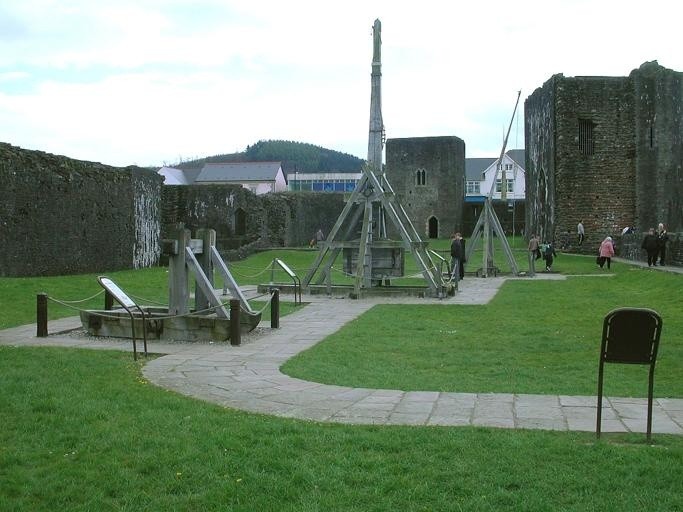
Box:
[456,232,466,280]
[641,228,659,267]
[529,234,556,272]
[656,222,668,265]
[609,236,617,255]
[450,235,461,281]
[599,236,614,270]
[316,229,324,248]
[577,219,585,245]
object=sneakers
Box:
[546,267,551,272]
